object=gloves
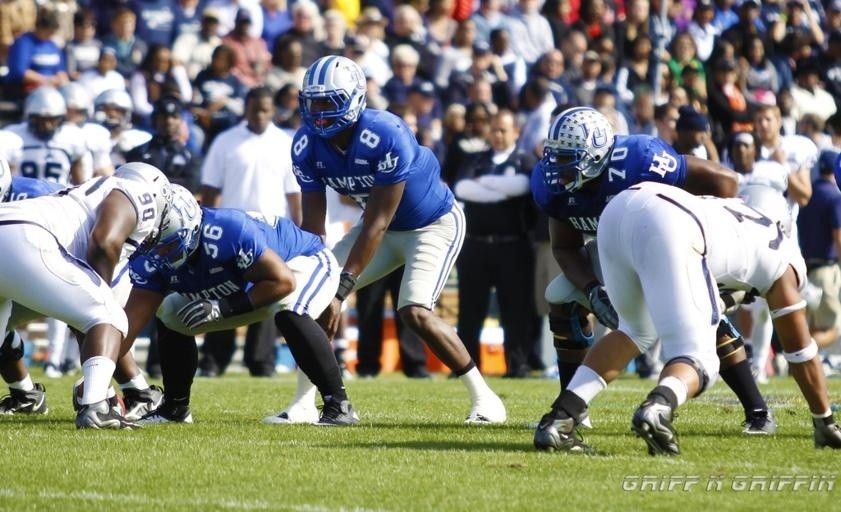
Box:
[813,415,841,449]
[539,107,615,192]
[177,299,233,329]
[585,281,618,331]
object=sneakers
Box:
[74,385,192,430]
[464,402,507,424]
[740,409,773,434]
[266,396,359,425]
[631,399,679,455]
[534,413,588,454]
[0,383,48,415]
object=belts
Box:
[625,187,706,256]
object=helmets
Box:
[113,162,203,274]
[739,184,790,238]
[25,84,135,115]
[298,55,367,136]
